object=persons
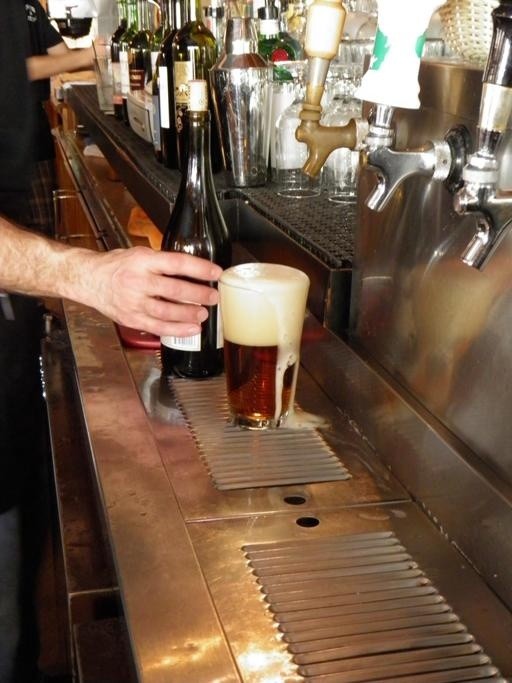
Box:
[0,0,110,182]
[0,1,224,682]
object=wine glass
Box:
[274,56,326,198]
[324,61,371,206]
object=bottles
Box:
[149,387,214,502]
[156,77,230,378]
[110,0,307,187]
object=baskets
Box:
[438,1,496,68]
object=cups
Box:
[92,55,115,115]
[219,261,309,430]
